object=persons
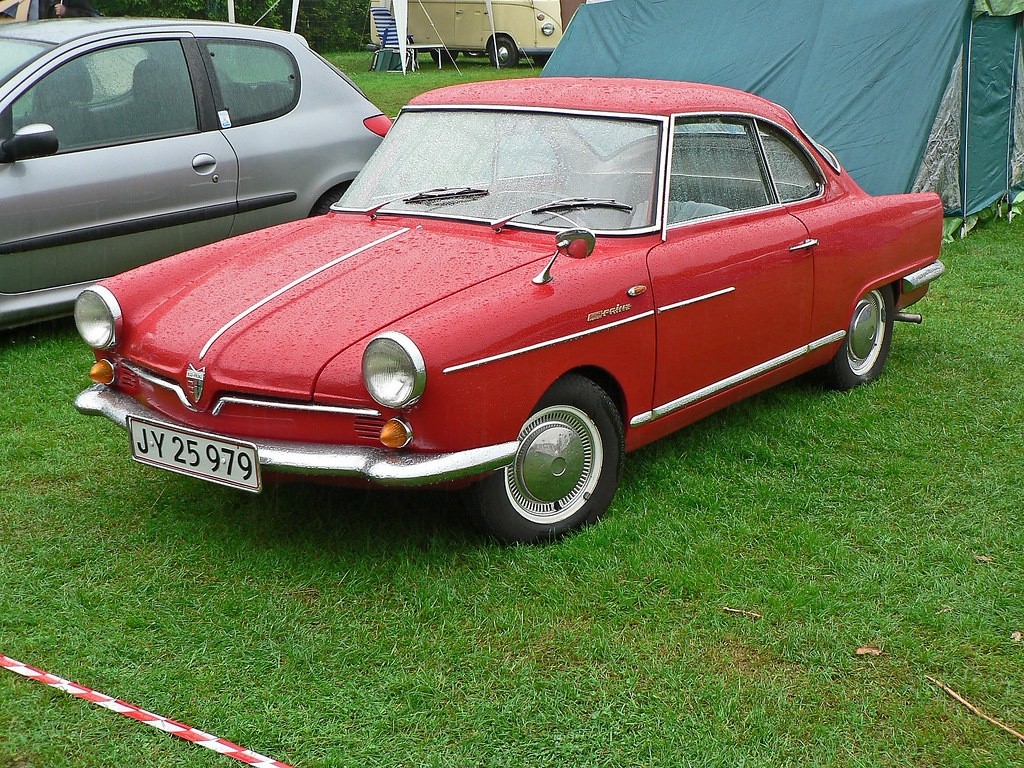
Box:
[48,0,94,19]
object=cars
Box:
[370,0,587,68]
[0,18,393,329]
[74,78,946,545]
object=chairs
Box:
[606,200,731,231]
[17,59,94,150]
[130,59,175,132]
[365,6,444,73]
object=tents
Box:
[535,0,1024,248]
